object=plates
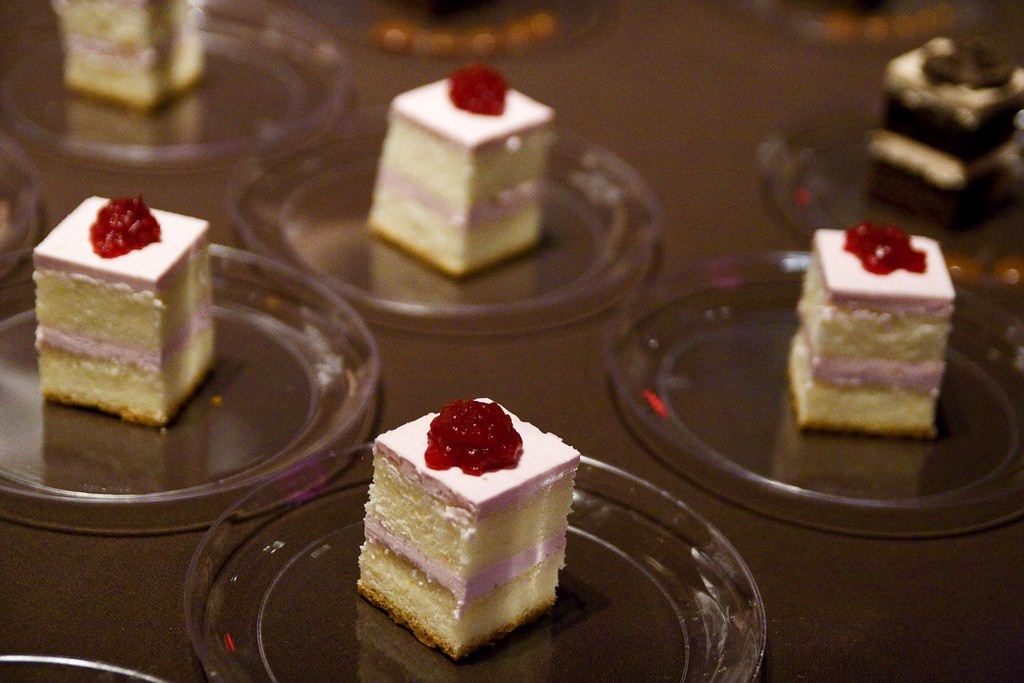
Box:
[0,0,349,166]
[183,442,767,683]
[607,251,1024,539]
[0,133,38,279]
[762,115,1024,302]
[0,241,377,534]
[287,0,609,58]
[230,106,659,336]
[726,0,998,47]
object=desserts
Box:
[31,193,217,427]
[868,31,1024,233]
[787,221,955,439]
[368,59,556,276]
[357,397,581,660]
[51,0,206,108]
